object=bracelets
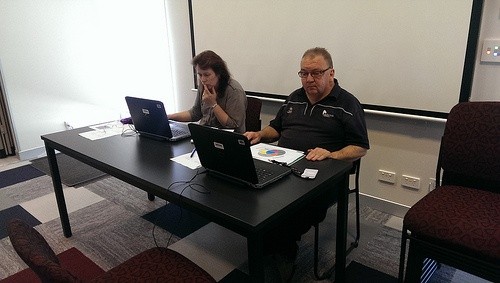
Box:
[210,104,219,110]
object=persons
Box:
[167,50,247,134]
[243,49,370,283]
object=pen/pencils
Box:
[190,147,196,158]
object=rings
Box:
[203,94,205,96]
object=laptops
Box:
[188,123,292,188]
[125,95,190,142]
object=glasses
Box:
[298,67,331,78]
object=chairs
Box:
[243,96,360,281]
[399,102,500,283]
[7,217,217,283]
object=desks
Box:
[40,117,355,283]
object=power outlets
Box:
[479,39,500,64]
[429,177,442,193]
[377,170,396,184]
[401,175,421,189]
